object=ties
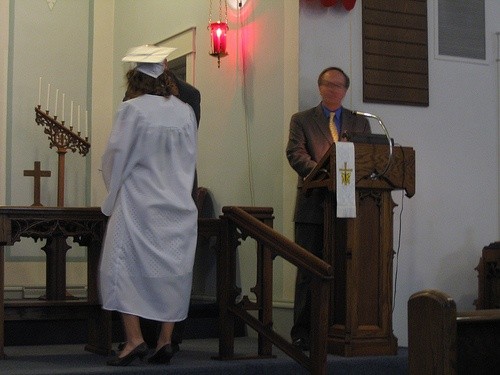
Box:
[329,111,338,142]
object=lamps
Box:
[205,0,230,69]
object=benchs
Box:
[405,289,500,375]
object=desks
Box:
[0,204,115,355]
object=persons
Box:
[285,66,371,351]
[97,45,202,365]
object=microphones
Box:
[352,111,393,179]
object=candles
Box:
[78,105,81,131]
[54,89,58,116]
[46,82,51,110]
[85,110,88,137]
[62,93,66,121]
[38,76,42,104]
[70,100,74,126]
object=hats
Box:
[121,44,177,79]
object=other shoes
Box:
[117,342,125,351]
[170,343,179,353]
[294,338,307,350]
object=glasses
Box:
[320,80,345,89]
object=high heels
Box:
[107,342,147,367]
[146,343,173,365]
